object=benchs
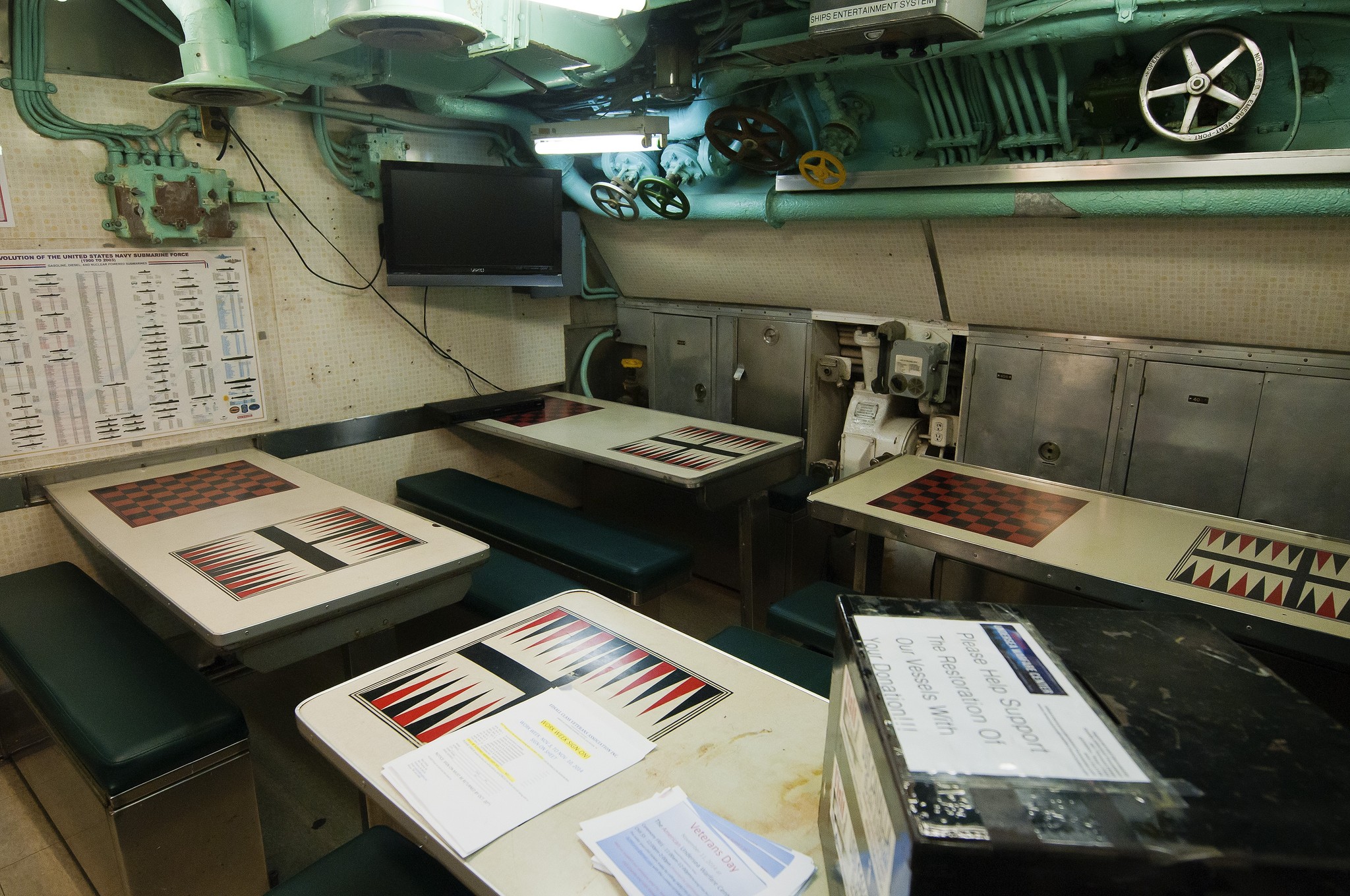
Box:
[394,467,687,629]
[0,558,271,894]
[701,624,832,699]
[255,824,477,895]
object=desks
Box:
[806,453,1348,722]
[42,449,489,872]
[295,588,837,896]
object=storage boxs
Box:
[814,594,1349,894]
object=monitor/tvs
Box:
[380,160,565,286]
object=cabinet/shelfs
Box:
[1123,358,1350,544]
[956,334,1118,489]
[616,295,847,480]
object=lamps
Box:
[530,116,669,154]
[528,0,647,19]
[650,37,702,102]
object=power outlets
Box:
[199,105,231,143]
[930,415,954,447]
